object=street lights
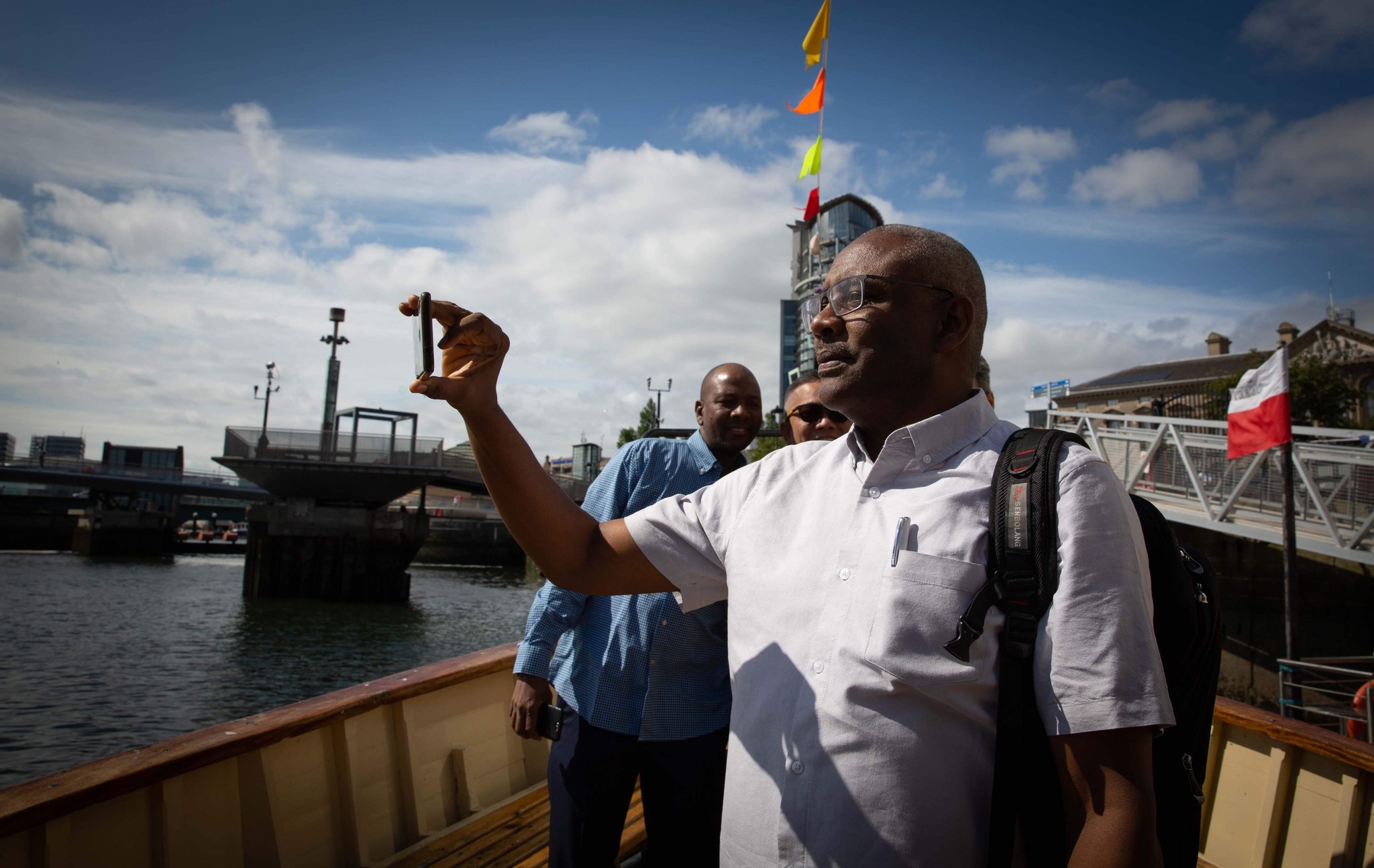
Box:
[321,308,349,461]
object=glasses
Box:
[801,274,957,334]
[784,402,848,423]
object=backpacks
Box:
[945,428,1228,868]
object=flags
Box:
[1226,345,1293,460]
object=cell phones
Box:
[412,292,435,380]
[535,703,563,742]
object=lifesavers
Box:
[1347,680,1374,740]
[109,495,129,506]
[435,509,442,517]
[125,472,147,477]
[136,498,152,512]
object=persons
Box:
[972,355,995,411]
[508,363,764,868]
[396,221,1176,868]
[778,369,854,445]
